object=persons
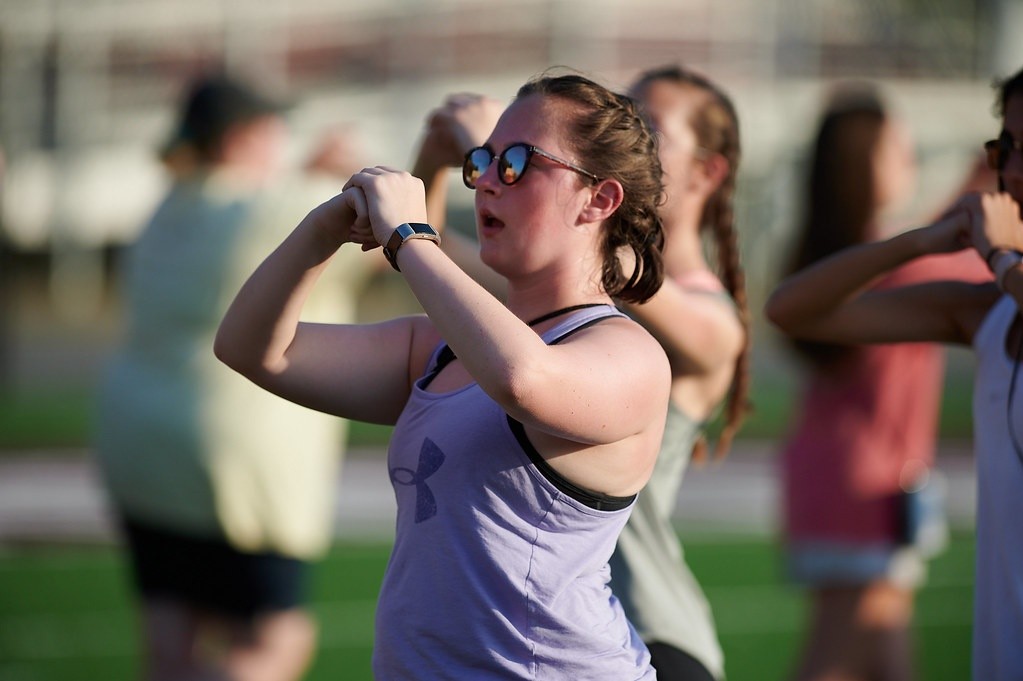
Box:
[211,74,673,681]
[777,89,995,681]
[97,77,366,681]
[765,71,1023,681]
[412,64,756,681]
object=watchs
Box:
[382,222,441,272]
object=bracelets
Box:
[986,245,1023,291]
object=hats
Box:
[156,71,299,159]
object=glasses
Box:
[462,142,606,190]
